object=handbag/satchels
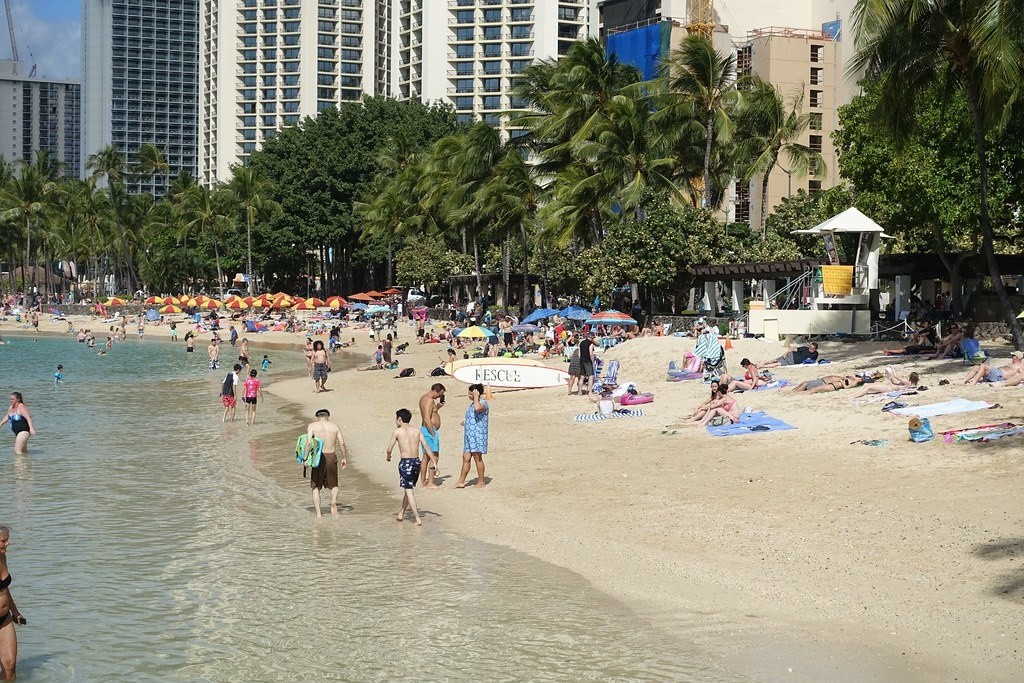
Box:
[909,416,934,442]
[326,366,331,372]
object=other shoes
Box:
[939,378,950,385]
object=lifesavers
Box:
[627,392,654,404]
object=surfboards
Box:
[452,363,570,387]
[294,434,324,468]
[444,357,546,375]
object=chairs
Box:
[592,358,622,391]
[961,339,989,365]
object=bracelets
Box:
[303,459,307,462]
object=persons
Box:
[689,380,740,427]
[105,307,355,425]
[0,283,105,355]
[0,526,26,683]
[754,294,761,301]
[719,358,772,392]
[386,408,438,526]
[0,392,35,454]
[848,364,920,399]
[519,319,638,397]
[351,297,518,371]
[757,343,819,369]
[639,315,725,382]
[883,320,963,360]
[961,351,1024,387]
[419,383,447,489]
[885,289,953,321]
[727,318,745,339]
[774,296,799,310]
[787,371,884,394]
[301,409,347,519]
[53,364,63,384]
[451,383,489,489]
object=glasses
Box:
[742,364,745,367]
[950,327,958,329]
[1012,354,1015,357]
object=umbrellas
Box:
[453,325,495,358]
[511,306,637,346]
[103,287,403,313]
[411,304,428,312]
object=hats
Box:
[909,418,922,430]
[1010,350,1023,359]
[772,300,776,305]
[588,332,595,338]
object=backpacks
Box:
[400,367,415,377]
[431,367,444,375]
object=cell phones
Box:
[440,395,445,403]
